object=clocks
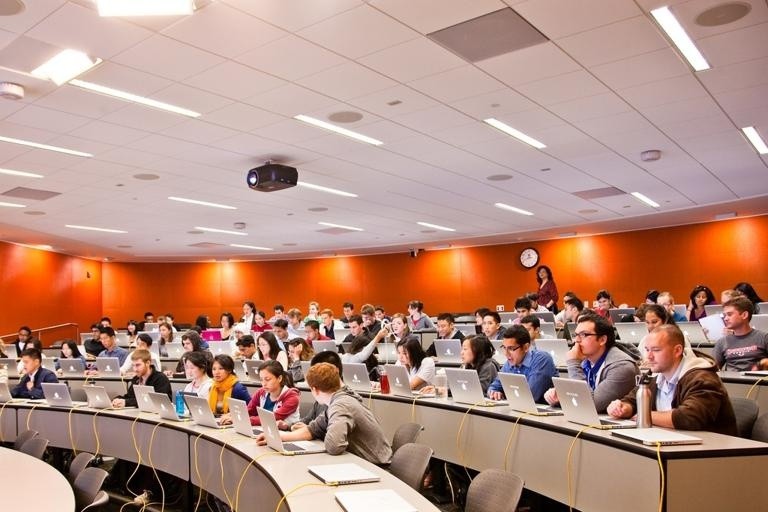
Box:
[519,247,540,270]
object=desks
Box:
[0,344,768,512]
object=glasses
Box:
[499,344,522,352]
[570,331,597,338]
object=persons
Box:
[54,300,363,487]
[488,325,560,405]
[1,326,59,400]
[535,267,559,315]
[307,362,393,468]
[712,283,768,372]
[513,285,715,366]
[607,325,738,437]
[340,299,508,486]
[543,314,641,414]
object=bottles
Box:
[635,373,653,428]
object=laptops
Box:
[132,385,159,413]
[296,329,308,340]
[208,341,231,359]
[226,397,287,439]
[609,428,702,446]
[202,330,222,342]
[145,322,160,331]
[445,367,508,407]
[498,372,564,416]
[307,463,380,484]
[151,359,173,378]
[342,341,353,353]
[41,359,55,372]
[312,340,337,356]
[478,302,768,369]
[41,382,88,408]
[59,358,97,376]
[245,359,265,382]
[232,361,247,380]
[552,377,638,429]
[334,490,419,512]
[2,383,24,404]
[2,357,25,379]
[319,328,325,336]
[166,342,184,357]
[300,361,311,374]
[184,394,234,429]
[383,363,435,398]
[434,338,463,363]
[116,333,135,347]
[342,363,381,393]
[95,356,126,377]
[79,333,94,344]
[377,342,399,361]
[454,326,477,337]
[255,405,323,453]
[150,342,159,357]
[334,328,351,346]
[77,344,88,360]
[148,391,193,422]
[2,343,18,358]
[82,384,136,409]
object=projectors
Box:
[247,164,298,192]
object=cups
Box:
[434,375,447,400]
[175,389,184,416]
[380,371,390,394]
